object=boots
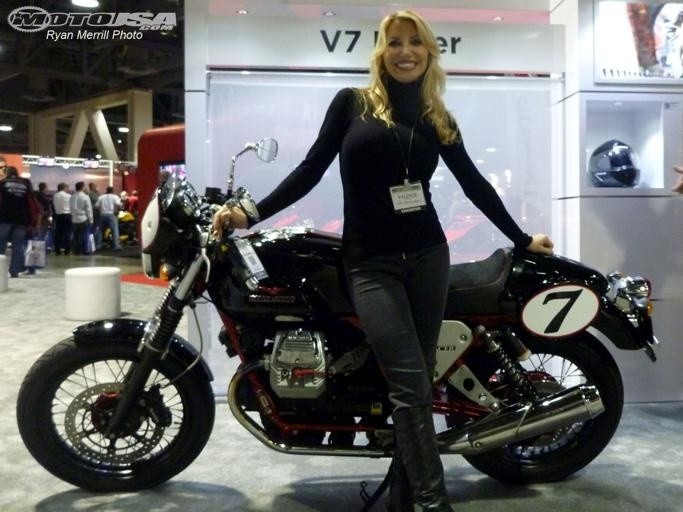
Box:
[385,434,416,512]
[392,405,455,512]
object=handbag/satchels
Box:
[25,235,47,268]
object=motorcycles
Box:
[16,137,662,512]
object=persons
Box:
[0,155,142,279]
[209,11,555,511]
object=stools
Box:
[62,265,120,323]
